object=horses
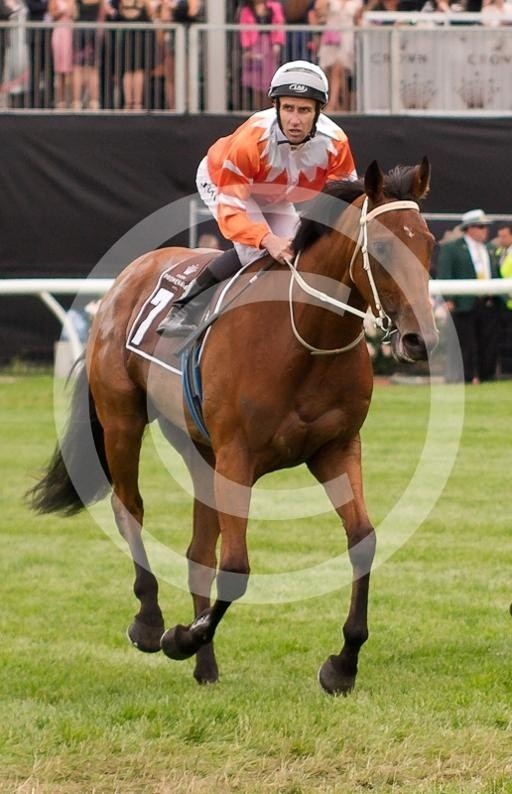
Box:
[20,155,440,699]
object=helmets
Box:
[266,56,332,106]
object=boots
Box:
[154,266,222,339]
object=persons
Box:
[1,0,512,115]
[490,222,512,379]
[437,208,496,383]
[154,59,358,337]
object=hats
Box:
[457,207,496,231]
